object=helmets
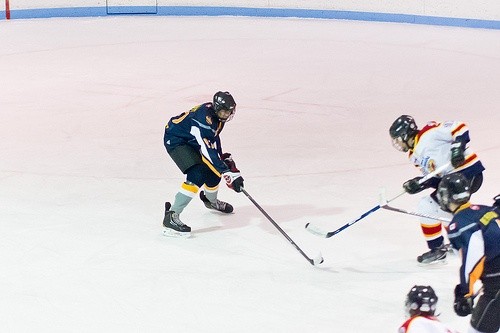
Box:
[388,114,418,142]
[408,285,438,313]
[213,91,236,113]
[436,173,470,211]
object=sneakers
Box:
[199,190,235,215]
[417,243,448,269]
[162,202,192,238]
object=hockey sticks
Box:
[239,186,324,266]
[305,153,470,239]
[379,186,453,223]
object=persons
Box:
[438,172,500,333]
[163,91,244,239]
[389,115,485,269]
[398,285,452,333]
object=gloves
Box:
[403,176,430,194]
[223,171,244,193]
[453,285,474,316]
[221,152,240,173]
[450,147,465,167]
